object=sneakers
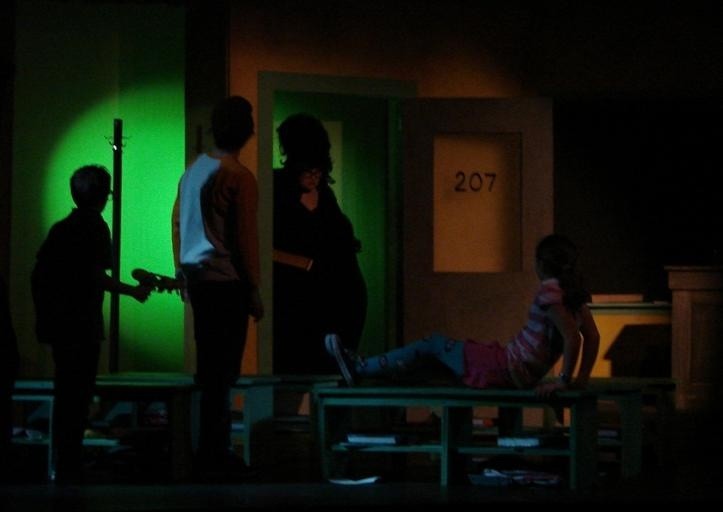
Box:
[325,333,360,387]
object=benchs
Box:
[0,372,680,491]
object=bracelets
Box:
[242,280,260,289]
[558,369,571,381]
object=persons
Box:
[266,151,367,486]
[31,164,149,473]
[165,95,264,473]
[325,232,600,396]
